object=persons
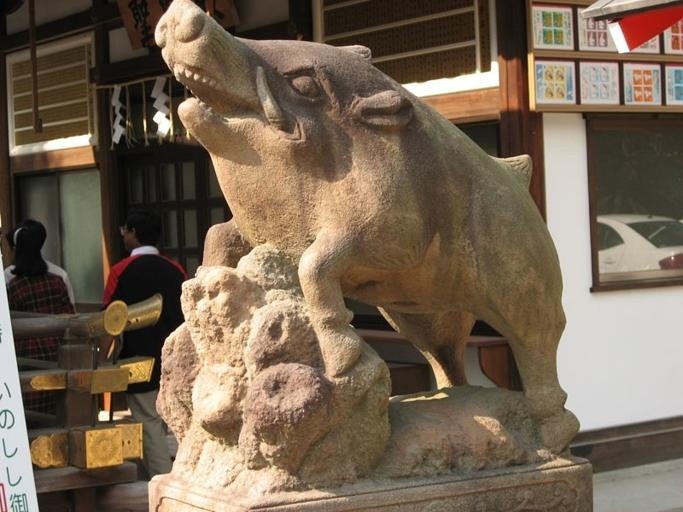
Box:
[4,226,75,428]
[2,218,74,315]
[100,212,188,480]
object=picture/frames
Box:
[526,2,683,115]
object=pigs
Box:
[153,0,580,457]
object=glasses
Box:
[119,225,131,234]
